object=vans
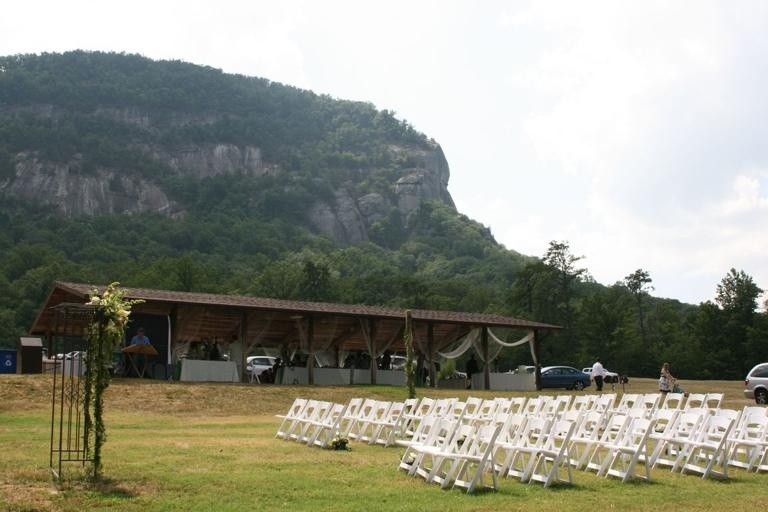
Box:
[744,361,768,404]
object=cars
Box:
[581,366,619,385]
[536,365,592,391]
[379,356,419,376]
[243,354,283,381]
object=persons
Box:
[262,342,392,389]
[659,363,675,393]
[466,353,478,390]
[210,344,221,360]
[130,327,152,377]
[189,348,201,360]
[591,356,605,391]
